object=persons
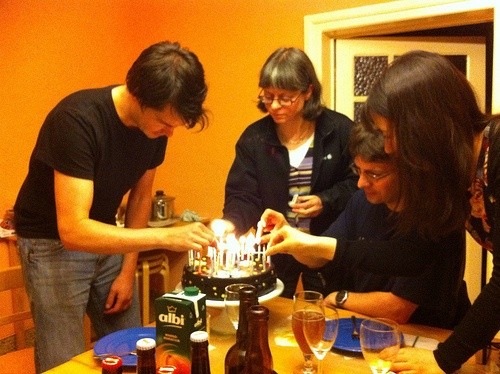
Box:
[264,50,500,374]
[260,122,472,331]
[13,40,216,374]
[209,47,357,304]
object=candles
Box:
[189,219,271,276]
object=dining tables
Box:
[40,293,500,374]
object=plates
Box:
[95,327,157,366]
[148,218,176,227]
[0,218,16,238]
[173,278,285,307]
[325,317,404,352]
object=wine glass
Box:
[292,291,325,373]
[302,303,338,373]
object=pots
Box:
[149,190,176,222]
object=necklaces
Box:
[278,128,309,145]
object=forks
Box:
[350,315,360,344]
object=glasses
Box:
[258,86,306,107]
[349,160,399,185]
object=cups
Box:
[359,317,401,373]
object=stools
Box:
[134,251,170,327]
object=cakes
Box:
[181,252,276,301]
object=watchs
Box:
[336,290,347,308]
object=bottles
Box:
[224,285,258,374]
[101,355,123,374]
[243,304,274,374]
[136,337,157,374]
[190,330,212,374]
